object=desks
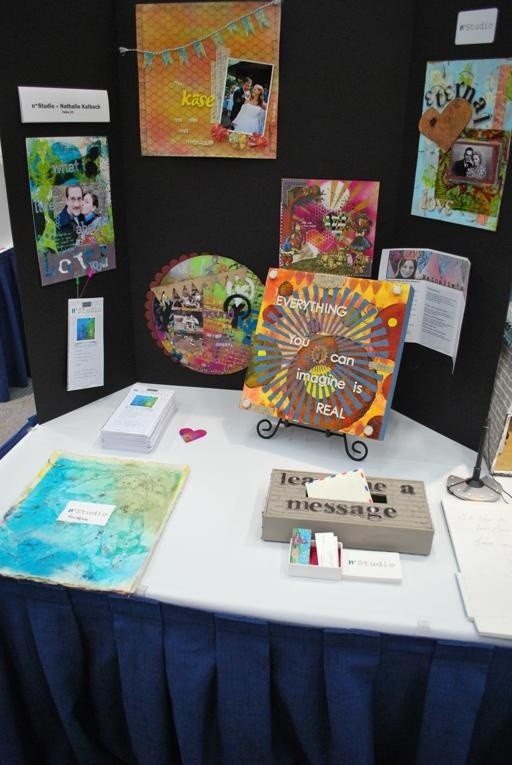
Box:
[0,381,512,764]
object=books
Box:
[0,452,189,598]
[99,381,179,454]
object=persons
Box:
[452,145,473,175]
[52,182,86,244]
[80,191,99,224]
[463,152,486,180]
[395,258,417,279]
[228,76,269,135]
